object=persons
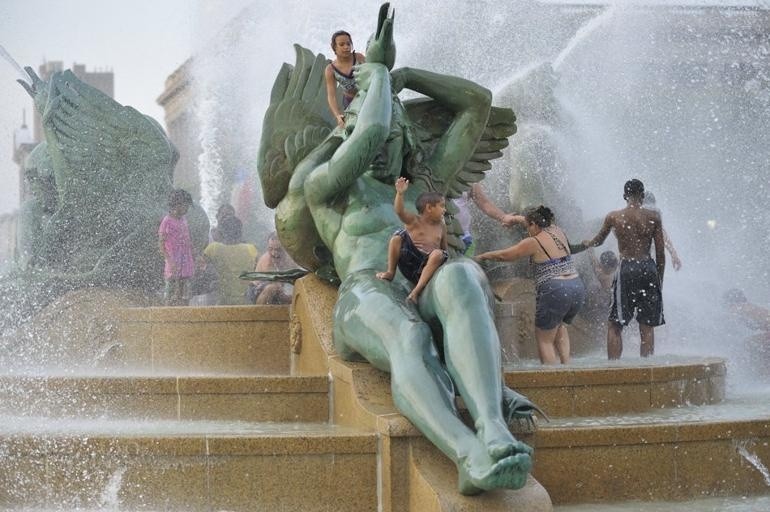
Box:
[300,58,536,498]
[199,204,300,305]
[156,187,199,307]
[324,31,368,128]
[451,179,520,254]
[470,206,587,366]
[374,176,450,306]
[569,180,770,382]
